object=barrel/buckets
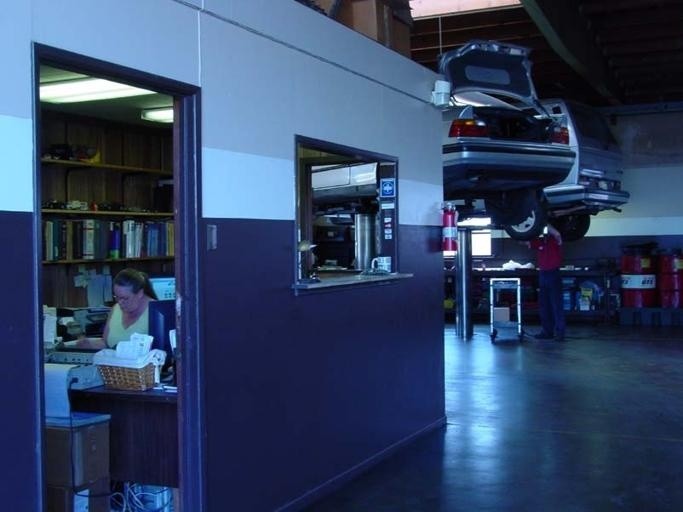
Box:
[620,254,657,306]
[653,248,683,308]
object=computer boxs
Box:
[134,485,170,512]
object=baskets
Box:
[96,363,155,391]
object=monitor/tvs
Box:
[147,299,176,363]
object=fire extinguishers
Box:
[440,201,459,251]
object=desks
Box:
[70,389,177,489]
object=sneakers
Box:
[534,333,564,341]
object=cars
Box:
[308,215,355,266]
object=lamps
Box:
[431,79,452,110]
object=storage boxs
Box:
[44,411,110,512]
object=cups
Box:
[371,256,392,273]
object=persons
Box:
[519,223,565,341]
[75,269,158,350]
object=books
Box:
[41,217,174,262]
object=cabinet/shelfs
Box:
[489,275,527,341]
[40,102,175,303]
